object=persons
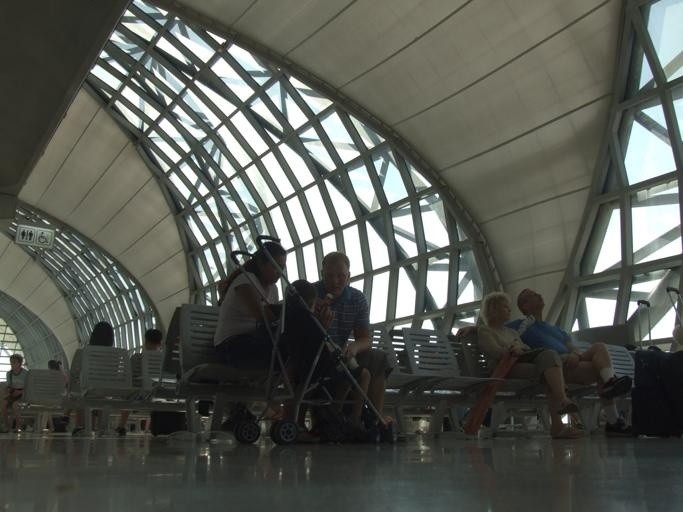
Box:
[71,321,116,438]
[115,329,163,436]
[0,354,30,433]
[455,287,633,438]
[311,252,394,445]
[46,358,69,432]
[211,241,347,446]
[477,290,587,440]
[269,278,372,441]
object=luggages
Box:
[631,287,682,440]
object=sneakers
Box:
[114,426,127,436]
[604,416,632,437]
[71,426,85,437]
[598,376,632,400]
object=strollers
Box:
[228,233,399,448]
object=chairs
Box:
[0,304,640,438]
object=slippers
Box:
[551,427,578,439]
[557,399,578,415]
